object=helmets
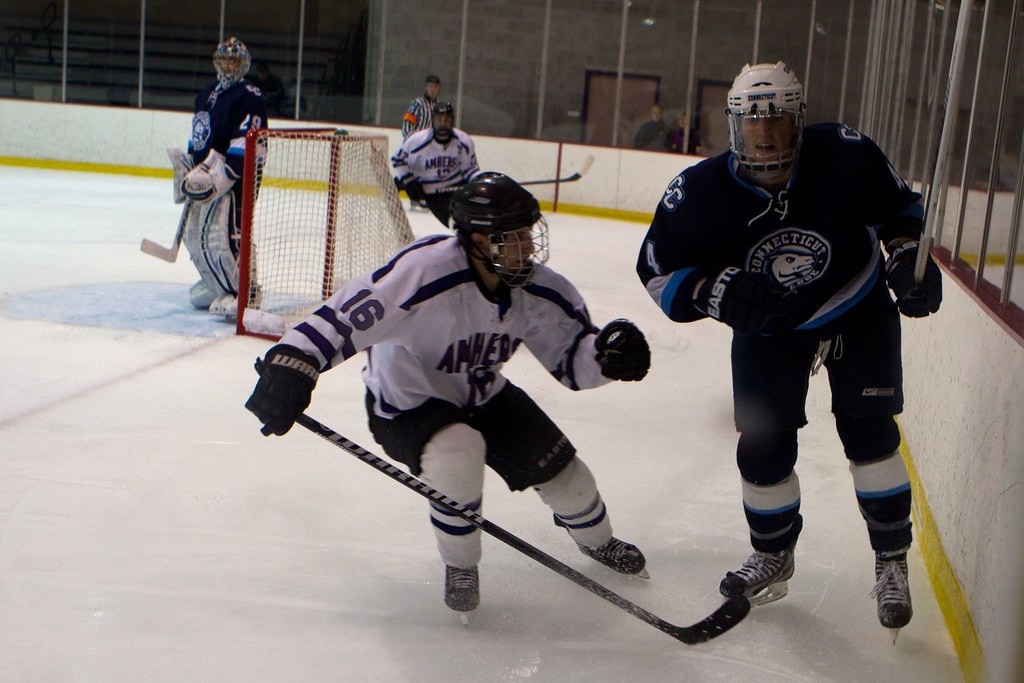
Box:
[431,101,453,117]
[213,36,250,87]
[725,60,806,179]
[448,172,550,287]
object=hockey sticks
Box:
[424,153,597,195]
[914,0,974,280]
[141,195,194,263]
[292,412,752,647]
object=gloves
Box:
[693,265,802,339]
[884,241,942,318]
[401,173,423,203]
[594,318,651,381]
[245,344,321,436]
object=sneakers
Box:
[867,544,914,646]
[719,545,795,607]
[444,564,480,626]
[575,535,651,581]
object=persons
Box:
[636,63,943,645]
[402,75,441,213]
[392,101,480,231]
[166,38,261,318]
[249,173,652,626]
[634,105,703,153]
